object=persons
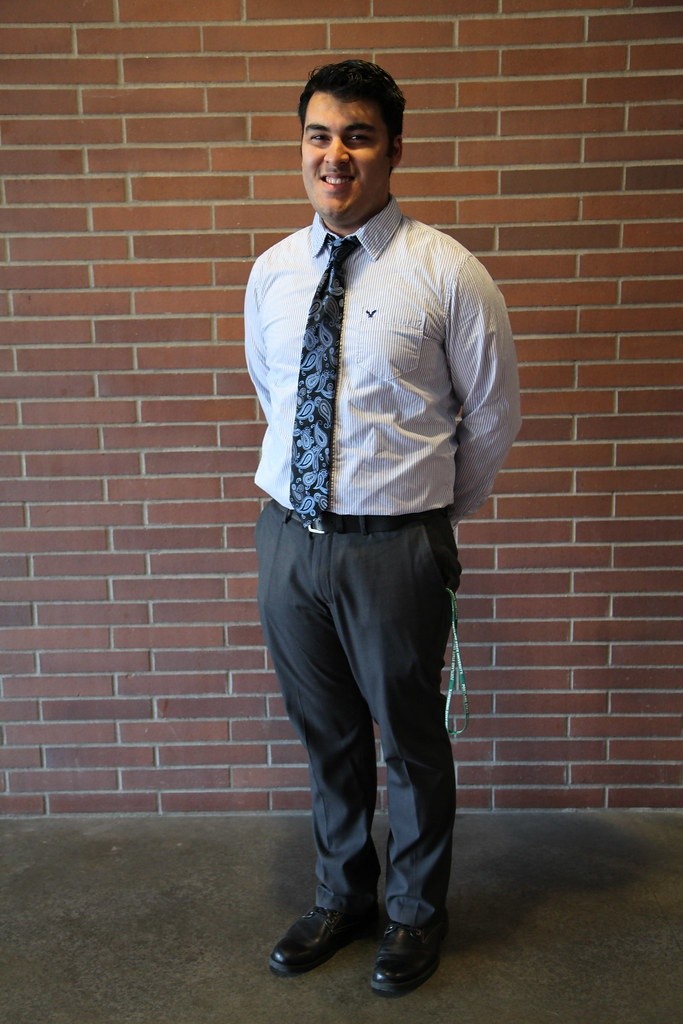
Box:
[244,60,521,995]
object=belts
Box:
[273,498,447,535]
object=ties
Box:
[288,234,360,530]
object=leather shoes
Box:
[371,906,449,993]
[270,902,379,973]
